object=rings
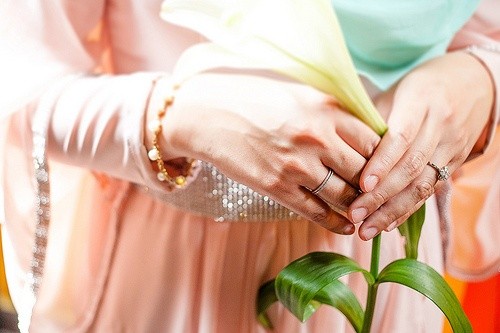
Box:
[427,161,450,181]
[310,169,334,195]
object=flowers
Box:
[158,0,474,333]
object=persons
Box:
[0,0,500,333]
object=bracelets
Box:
[148,75,199,189]
